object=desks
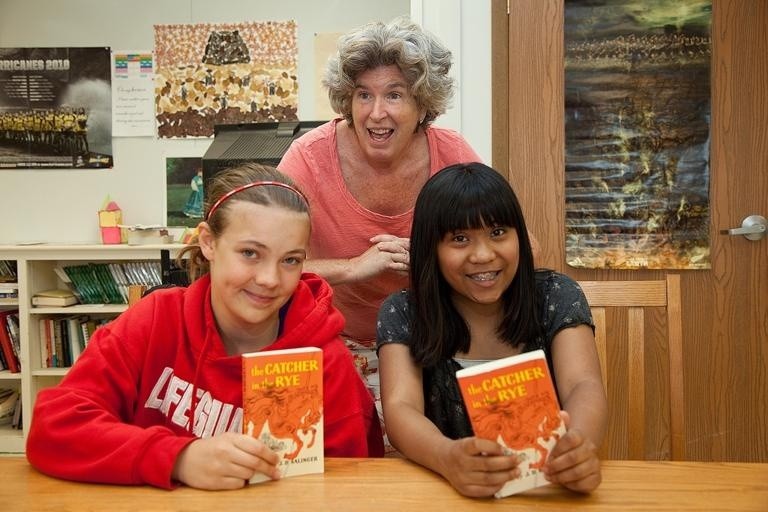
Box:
[0,451,768,510]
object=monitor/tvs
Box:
[200,120,330,216]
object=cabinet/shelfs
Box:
[0,241,207,456]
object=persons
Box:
[25,161,375,494]
[375,162,608,502]
[276,16,483,459]
[0,104,91,154]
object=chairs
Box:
[562,273,688,465]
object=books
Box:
[455,349,565,500]
[240,346,326,484]
[0,258,190,430]
